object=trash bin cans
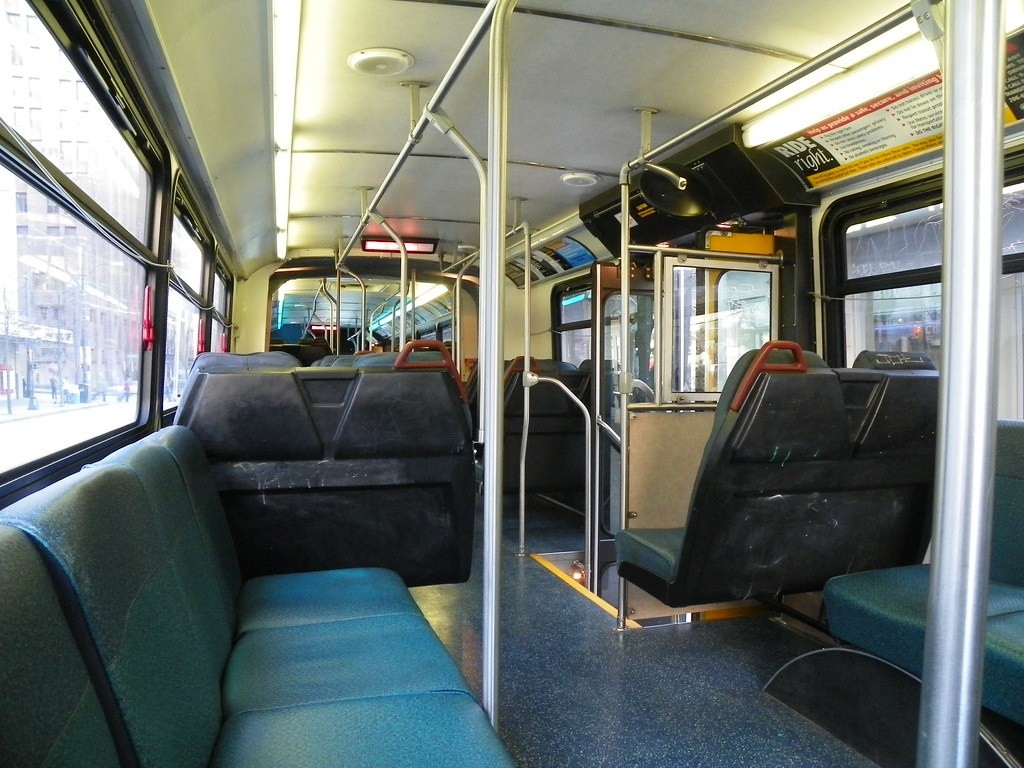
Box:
[79,383,89,403]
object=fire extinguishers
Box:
[570,560,585,586]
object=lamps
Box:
[739,0,1024,152]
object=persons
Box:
[124,379,129,402]
[50,375,57,399]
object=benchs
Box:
[0,324,1024,768]
